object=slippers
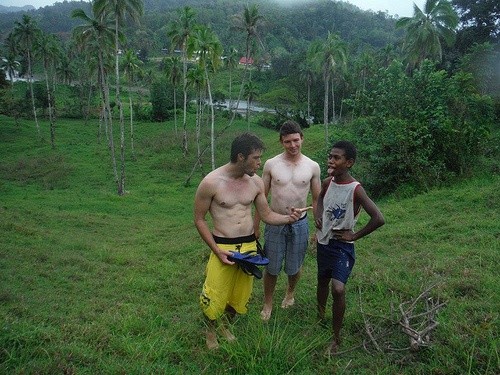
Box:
[229,250,270,266]
[228,257,263,280]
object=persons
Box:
[254,119,322,323]
[314,139,385,349]
[194,133,313,350]
